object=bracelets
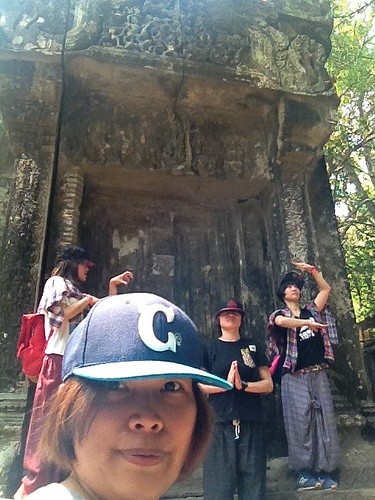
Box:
[87,295,94,305]
[310,268,315,276]
[241,381,248,392]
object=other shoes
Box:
[317,476,337,489]
[296,475,321,491]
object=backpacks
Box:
[17,311,54,383]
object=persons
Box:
[195,298,274,500]
[264,261,341,491]
[20,292,234,500]
[13,244,133,500]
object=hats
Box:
[215,297,246,318]
[57,246,96,266]
[279,272,305,286]
[61,292,233,395]
